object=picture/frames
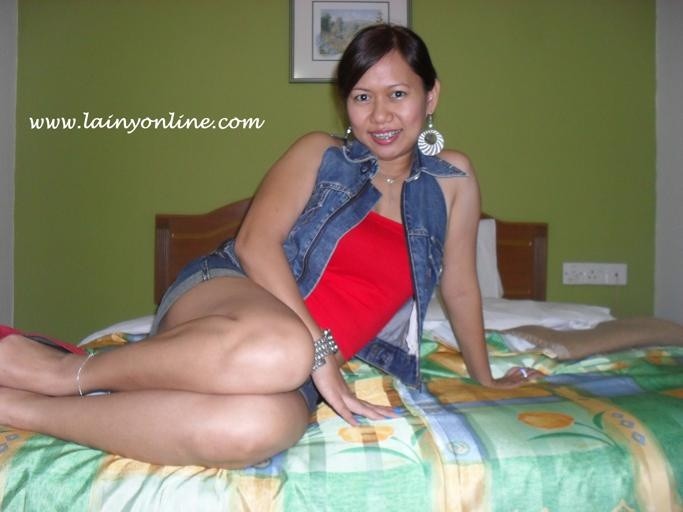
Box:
[287,0,414,83]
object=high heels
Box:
[0,325,87,358]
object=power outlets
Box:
[585,262,604,285]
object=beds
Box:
[0,197,682,511]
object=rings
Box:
[521,368,526,379]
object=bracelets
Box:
[310,324,341,374]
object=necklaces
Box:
[375,171,408,184]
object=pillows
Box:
[432,218,505,299]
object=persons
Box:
[0,22,548,472]
